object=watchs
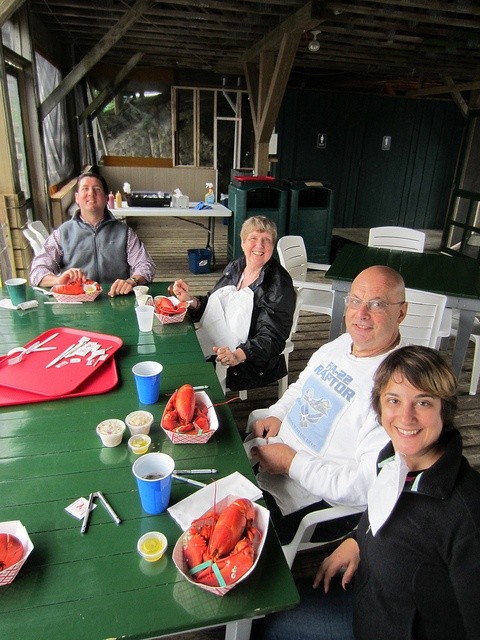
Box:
[128,277,139,286]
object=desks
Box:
[103,200,232,270]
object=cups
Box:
[132,453,175,515]
[132,362,163,405]
[5,278,27,306]
[134,306,155,332]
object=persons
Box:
[30,173,157,299]
[168,215,297,389]
[212,343,480,640]
[242,266,409,547]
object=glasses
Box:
[344,295,405,311]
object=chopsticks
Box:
[26,333,59,354]
[46,344,75,368]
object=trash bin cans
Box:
[187,248,213,275]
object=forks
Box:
[8,347,56,356]
[8,341,40,365]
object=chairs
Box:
[392,289,448,353]
[364,224,426,253]
[272,233,334,341]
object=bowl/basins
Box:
[125,411,154,436]
[133,286,149,296]
[128,434,152,455]
[138,531,168,564]
[95,419,126,448]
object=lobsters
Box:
[0,532,24,572]
[161,383,214,435]
[152,296,188,316]
[51,278,100,295]
[181,497,263,587]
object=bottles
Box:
[109,191,114,209]
[116,190,122,208]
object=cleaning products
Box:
[204,182,215,204]
